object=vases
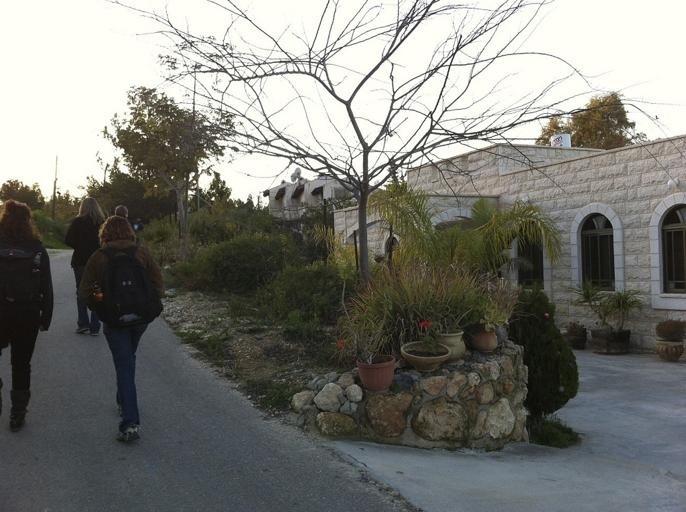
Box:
[570,338,587,350]
[401,343,450,373]
[439,267,467,358]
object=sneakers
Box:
[115,389,141,444]
[75,325,100,336]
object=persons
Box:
[64,198,165,441]
[0,199,53,432]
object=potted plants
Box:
[656,320,686,362]
[467,277,520,354]
[568,280,642,354]
[351,307,393,393]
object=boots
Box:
[9,388,31,433]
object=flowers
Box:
[420,320,438,353]
[565,322,587,336]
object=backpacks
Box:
[104,248,162,328]
[76,219,98,243]
[1,250,42,330]
